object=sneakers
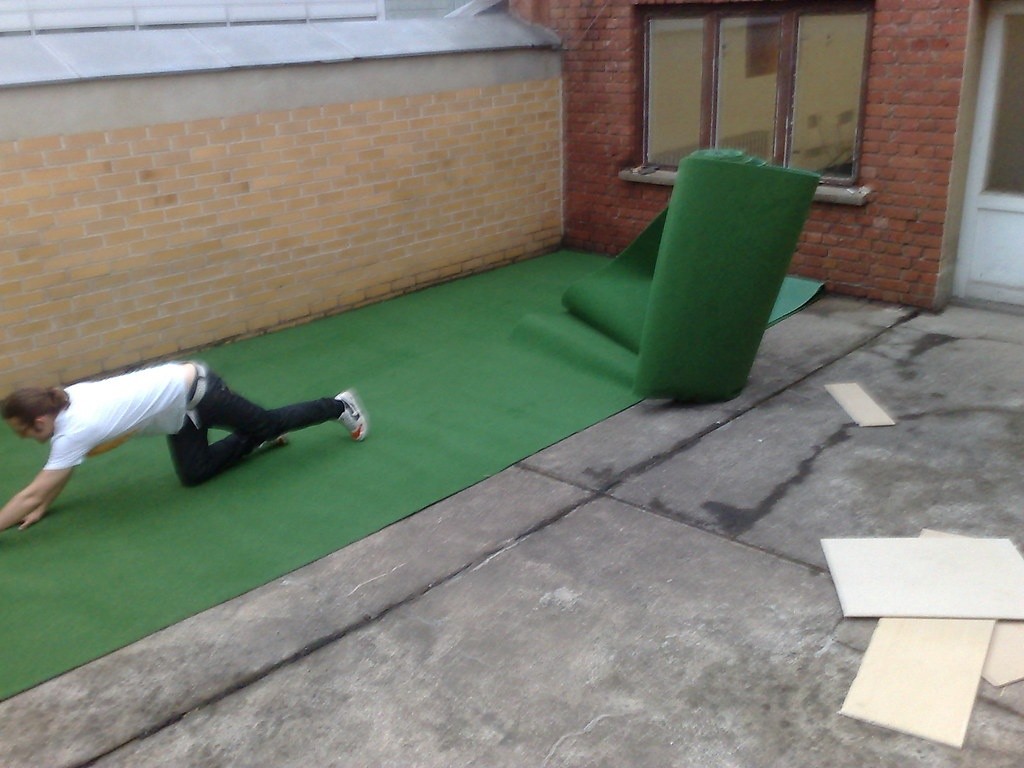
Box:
[272,436,287,446]
[335,392,368,442]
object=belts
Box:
[182,360,207,409]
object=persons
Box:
[0,361,366,532]
